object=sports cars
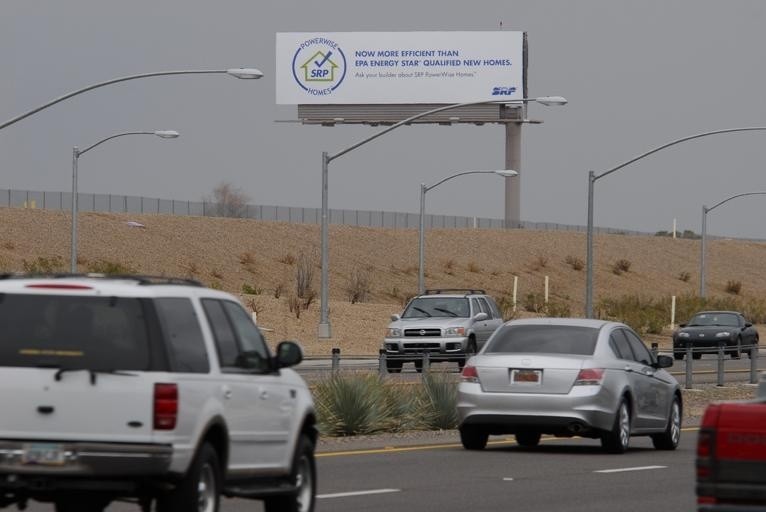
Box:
[672,310,760,362]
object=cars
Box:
[456,317,685,450]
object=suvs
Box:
[1,273,322,512]
[382,286,507,372]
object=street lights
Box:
[586,127,765,315]
[315,95,569,344]
[417,169,516,295]
[0,66,264,128]
[71,129,179,271]
